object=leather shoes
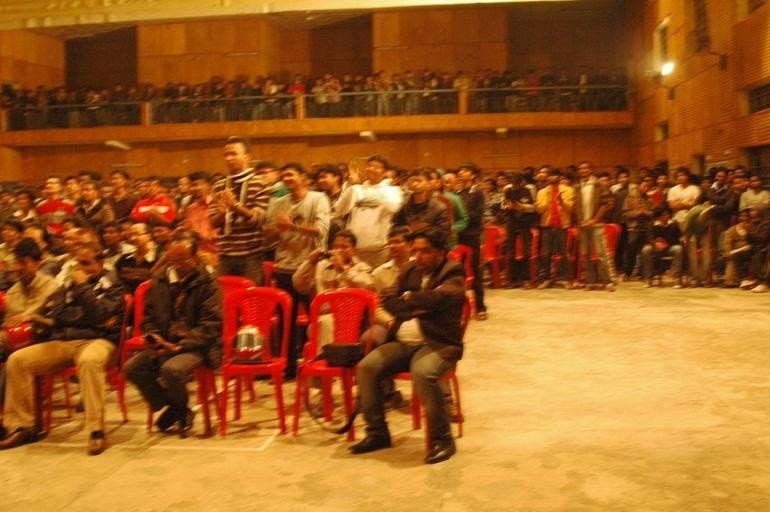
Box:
[424,437,458,464]
[0,428,38,450]
[347,432,392,455]
[87,431,105,455]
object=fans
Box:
[682,30,728,71]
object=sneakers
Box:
[751,285,768,292]
[179,407,197,437]
[739,279,758,288]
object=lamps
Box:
[651,62,678,100]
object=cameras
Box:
[319,251,331,258]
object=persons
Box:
[206,171,225,225]
[62,242,125,290]
[328,226,375,266]
[331,154,466,226]
[120,170,206,226]
[120,226,223,295]
[0,241,125,455]
[359,225,465,423]
[0,165,62,238]
[0,238,62,368]
[206,136,271,287]
[0,238,62,291]
[255,161,293,261]
[291,228,375,388]
[375,226,465,269]
[346,226,466,463]
[120,226,224,437]
[301,163,331,200]
[261,163,331,384]
[62,166,120,241]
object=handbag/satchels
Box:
[320,342,365,369]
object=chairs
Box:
[477,220,622,289]
[3,244,471,438]
